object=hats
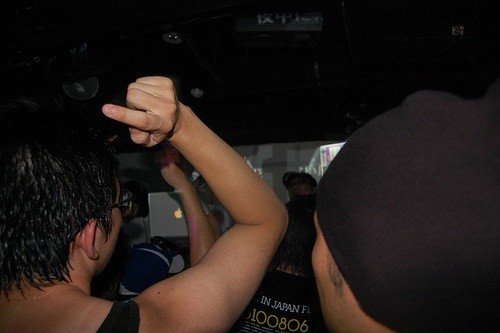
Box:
[282,171,316,188]
[120,242,184,293]
[317,91,500,333]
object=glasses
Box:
[76,190,133,234]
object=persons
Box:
[312,90,500,333]
[0,76,289,333]
[90,148,329,333]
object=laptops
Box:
[147,191,190,239]
